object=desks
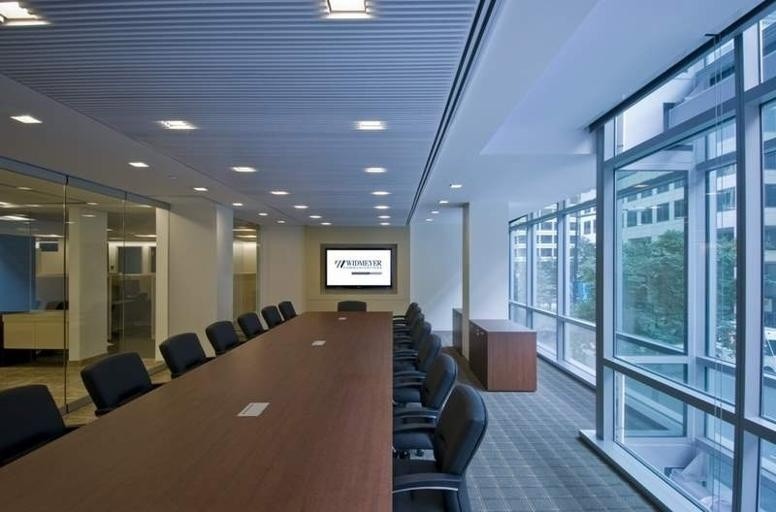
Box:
[469,319,538,392]
[0,301,393,511]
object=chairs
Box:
[393,301,489,512]
[159,332,216,379]
[278,301,298,321]
[1,383,84,468]
[237,312,269,340]
[336,301,367,312]
[205,320,247,356]
[80,352,168,417]
[260,305,284,328]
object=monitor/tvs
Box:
[325,248,393,289]
